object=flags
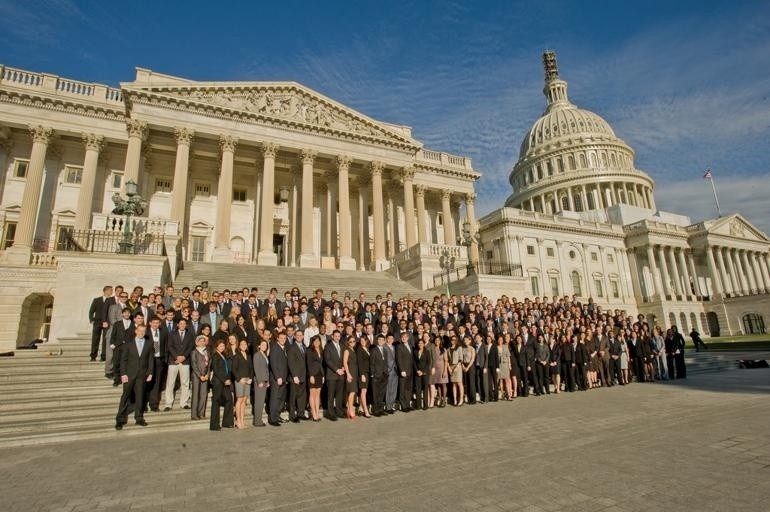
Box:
[704,170,711,179]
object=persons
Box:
[244,89,337,128]
[87,284,686,431]
[689,328,707,352]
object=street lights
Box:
[439,251,455,299]
[111,176,150,254]
[455,217,482,278]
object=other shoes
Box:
[135,421,148,426]
[105,374,112,379]
[466,387,560,405]
[113,381,121,386]
[184,406,191,410]
[101,356,106,361]
[636,371,686,383]
[373,398,463,417]
[143,407,148,411]
[192,410,371,431]
[164,407,172,412]
[151,408,160,412]
[115,423,122,431]
[91,358,96,361]
[563,377,629,392]
